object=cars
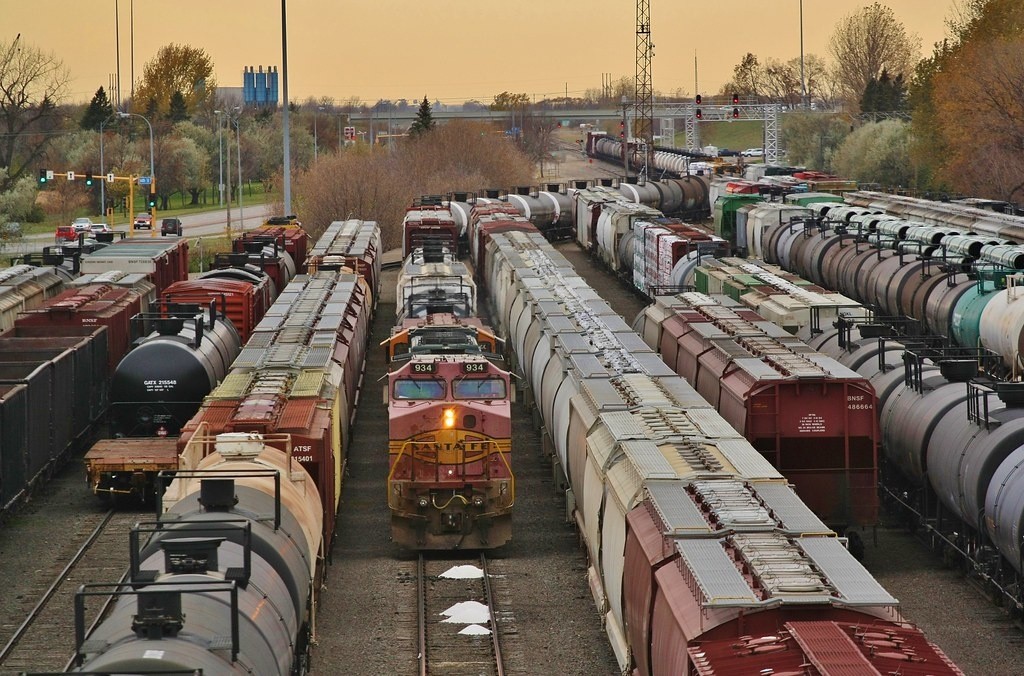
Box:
[718,148,738,157]
[71,218,92,231]
[55,226,79,242]
[745,148,763,157]
[87,224,111,239]
[133,213,152,229]
[741,149,754,156]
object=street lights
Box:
[370,100,389,145]
[215,110,244,232]
[319,106,342,155]
[120,113,157,239]
[219,106,241,206]
[389,98,405,148]
[100,111,122,222]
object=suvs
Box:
[161,219,183,236]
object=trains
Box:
[376,131,742,551]
[82,216,312,506]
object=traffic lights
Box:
[558,122,561,128]
[696,110,701,118]
[621,132,624,137]
[734,95,738,103]
[87,173,91,185]
[41,170,45,182]
[621,121,624,127]
[734,110,738,117]
[697,96,700,103]
[150,195,154,206]
